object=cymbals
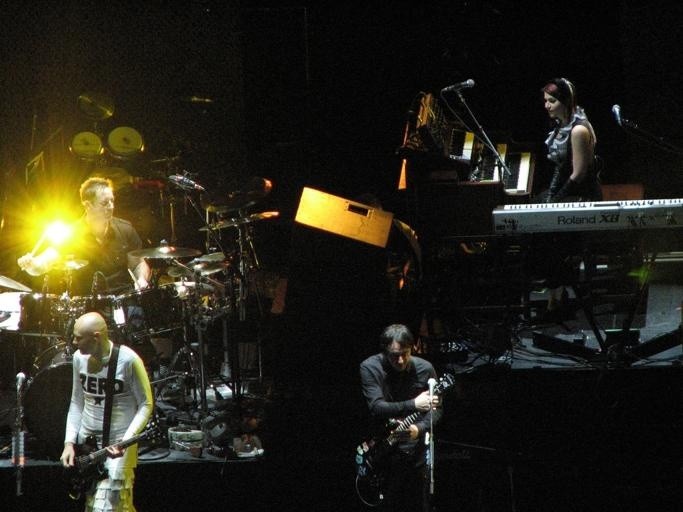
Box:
[74,89,116,122]
[51,259,89,272]
[199,175,271,215]
[126,245,202,261]
[197,212,280,232]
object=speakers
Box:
[293,184,396,250]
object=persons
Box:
[530,76,600,324]
[59,310,155,512]
[18,177,152,296]
[359,321,444,512]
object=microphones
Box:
[427,377,436,403]
[610,103,622,127]
[168,173,206,195]
[16,371,26,391]
[442,77,476,94]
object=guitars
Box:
[53,410,178,511]
[353,373,457,508]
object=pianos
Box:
[396,91,538,247]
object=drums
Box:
[0,274,32,294]
[65,130,102,171]
[114,285,199,341]
[33,292,113,340]
[105,126,146,165]
[21,342,155,456]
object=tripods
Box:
[152,299,224,408]
